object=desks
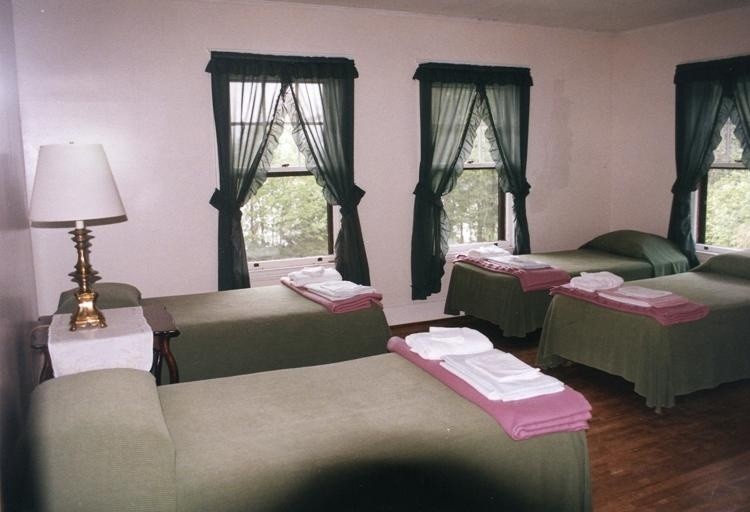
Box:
[29,305,181,385]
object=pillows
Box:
[694,253,749,276]
[56,282,140,311]
[25,368,177,512]
[578,232,685,264]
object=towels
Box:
[570,268,691,310]
[472,243,554,272]
[47,306,155,376]
[388,327,566,401]
[286,265,377,301]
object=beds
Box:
[22,354,591,512]
[28,279,395,385]
[534,253,749,412]
[441,231,695,340]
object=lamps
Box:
[29,140,123,330]
